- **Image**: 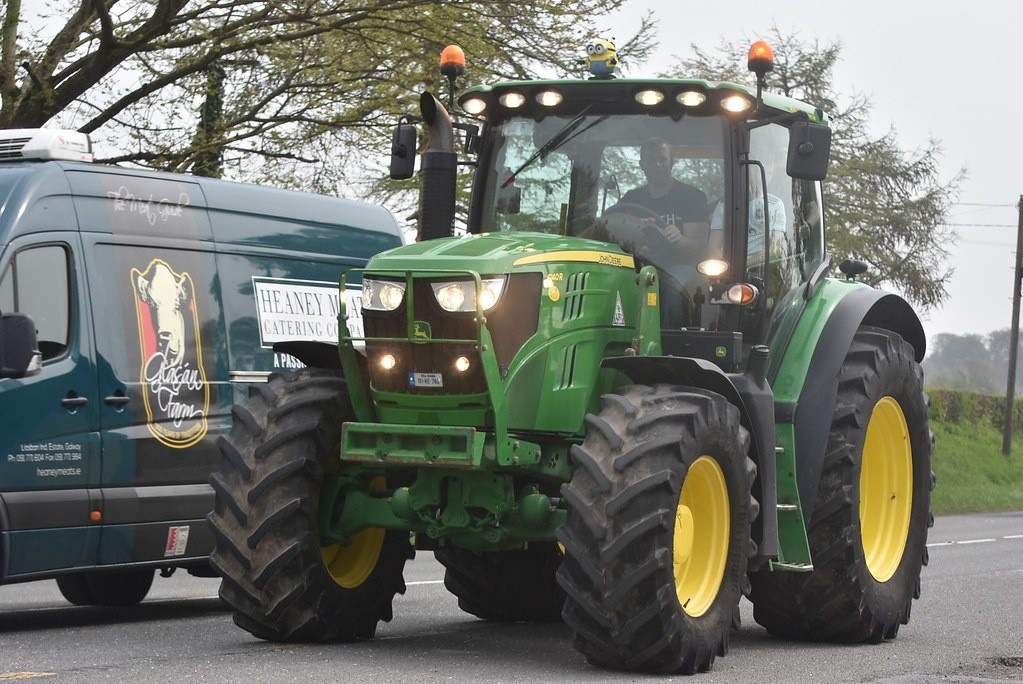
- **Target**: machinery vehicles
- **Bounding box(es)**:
[207,42,936,680]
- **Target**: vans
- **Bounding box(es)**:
[0,130,405,614]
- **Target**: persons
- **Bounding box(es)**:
[708,153,788,308]
[577,137,710,329]
